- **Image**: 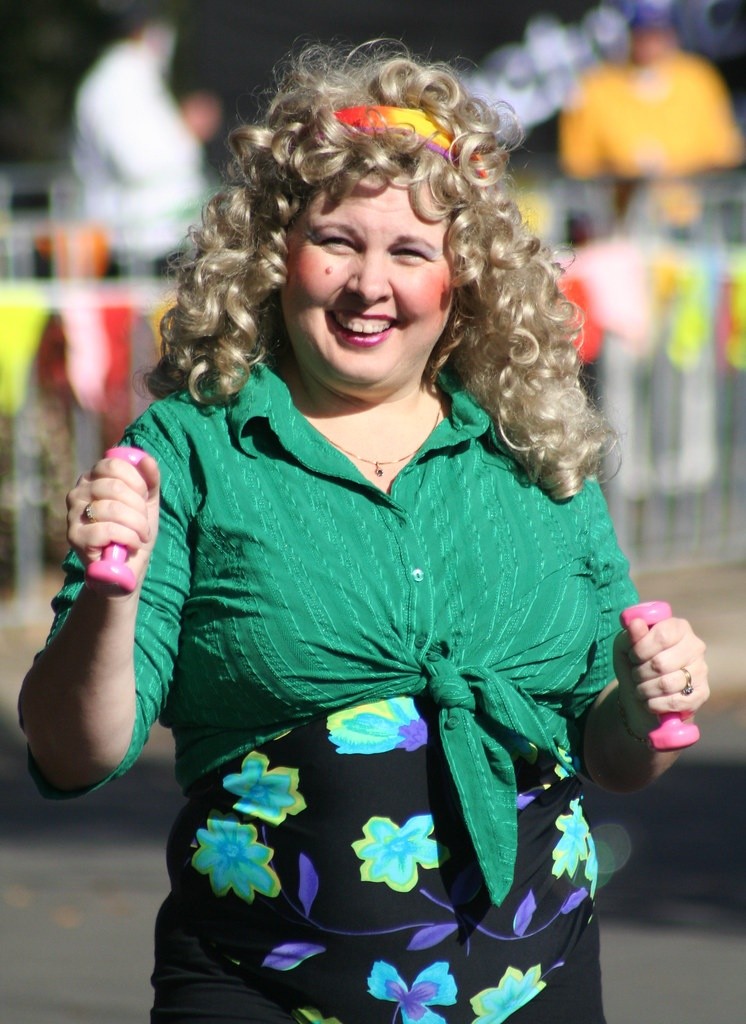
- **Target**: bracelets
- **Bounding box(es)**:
[617,695,648,742]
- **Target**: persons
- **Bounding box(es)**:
[17,39,711,1024]
[77,0,220,276]
[558,0,746,237]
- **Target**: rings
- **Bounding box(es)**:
[327,402,442,477]
[85,500,96,523]
[681,668,693,694]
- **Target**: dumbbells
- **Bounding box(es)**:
[82,443,155,600]
[620,595,705,752]
